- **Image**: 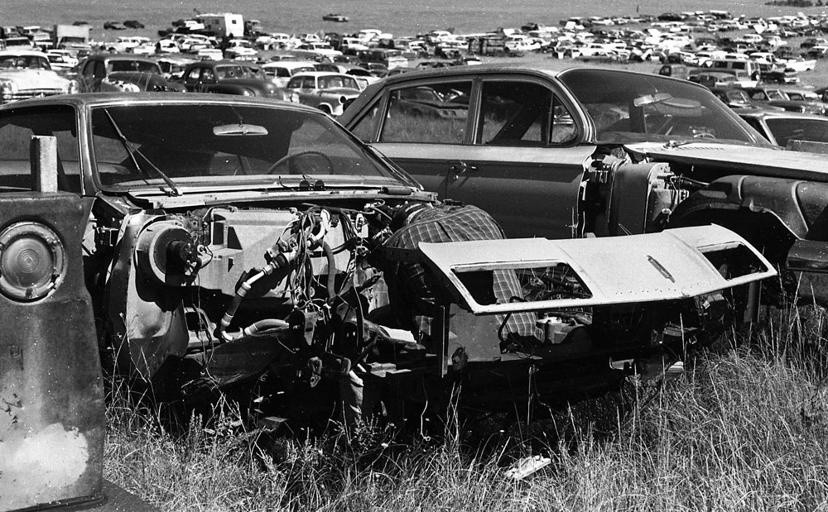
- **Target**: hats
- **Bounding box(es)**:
[389,202,427,234]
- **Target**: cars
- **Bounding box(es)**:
[339,66,825,300]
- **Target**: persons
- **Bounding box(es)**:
[375,198,539,366]
